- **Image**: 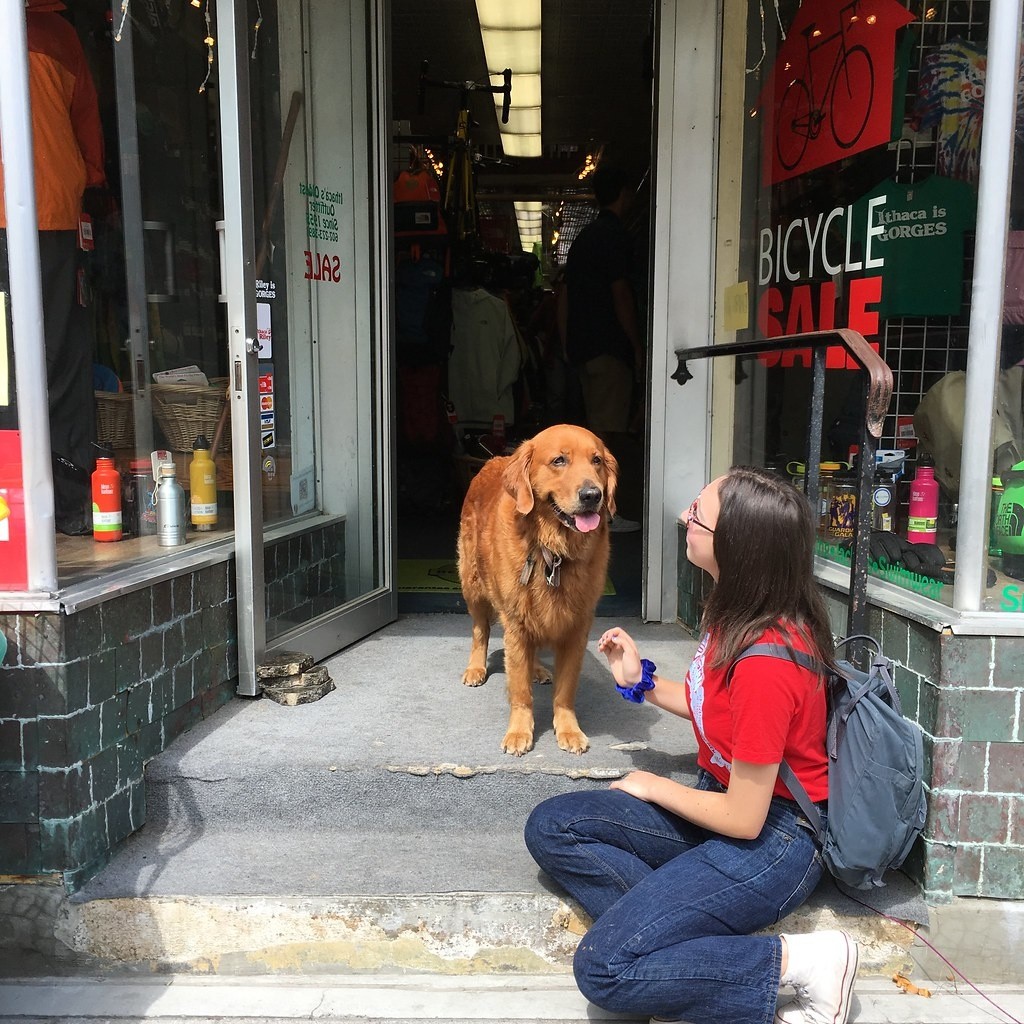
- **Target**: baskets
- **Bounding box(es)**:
[94,377,232,454]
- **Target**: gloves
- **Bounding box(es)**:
[839,530,946,575]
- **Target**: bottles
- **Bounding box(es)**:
[817,461,850,532]
[907,455,941,546]
[90,442,123,542]
[152,463,186,547]
[873,471,897,533]
[787,460,806,492]
[826,470,861,549]
[189,432,217,531]
[129,457,155,535]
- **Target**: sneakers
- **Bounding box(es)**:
[608,512,641,532]
[774,931,859,1024]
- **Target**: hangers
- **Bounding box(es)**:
[889,138,933,184]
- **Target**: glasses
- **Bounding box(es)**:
[686,486,714,533]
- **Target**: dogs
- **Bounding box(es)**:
[458,423,618,759]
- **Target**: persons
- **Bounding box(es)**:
[2,0,113,543]
[447,252,633,508]
[524,465,841,1024]
[566,169,638,533]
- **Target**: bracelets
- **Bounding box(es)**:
[615,660,655,703]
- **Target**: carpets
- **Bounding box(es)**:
[398,557,620,596]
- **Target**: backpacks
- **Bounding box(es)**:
[726,635,930,890]
[393,148,455,523]
